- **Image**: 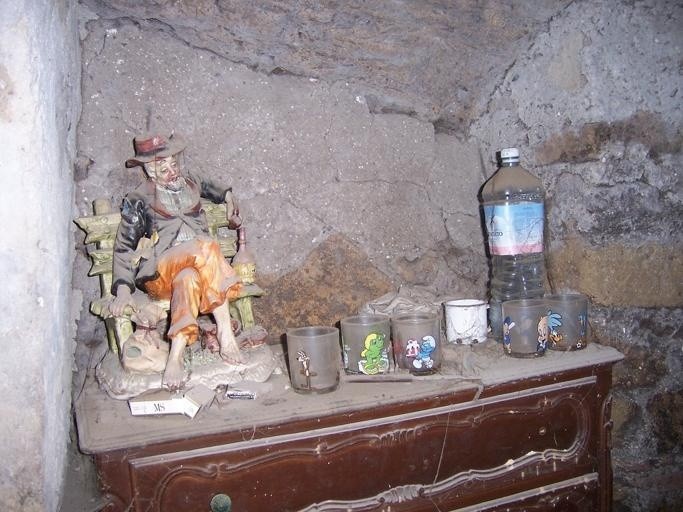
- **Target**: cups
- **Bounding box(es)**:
[502,299,548,358]
[441,298,492,346]
[285,325,341,394]
[393,313,443,376]
[338,315,392,375]
[544,293,589,353]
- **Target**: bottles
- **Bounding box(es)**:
[480,146,553,342]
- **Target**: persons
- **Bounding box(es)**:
[108,129,248,387]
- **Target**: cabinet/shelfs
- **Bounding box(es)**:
[76,340,626,512]
